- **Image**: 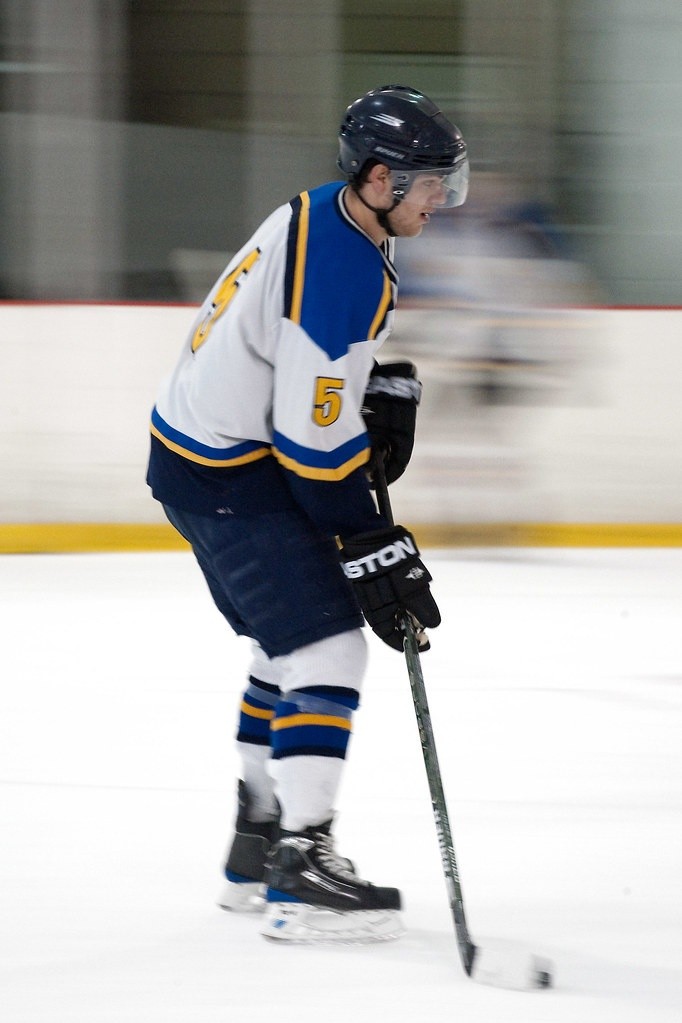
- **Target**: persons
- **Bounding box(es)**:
[146,84,471,949]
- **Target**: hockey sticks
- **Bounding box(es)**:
[370,454,553,996]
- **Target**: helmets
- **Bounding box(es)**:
[337,84,468,208]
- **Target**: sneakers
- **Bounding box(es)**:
[217,778,283,910]
[263,809,401,942]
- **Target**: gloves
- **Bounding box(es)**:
[361,359,422,490]
[337,525,441,653]
